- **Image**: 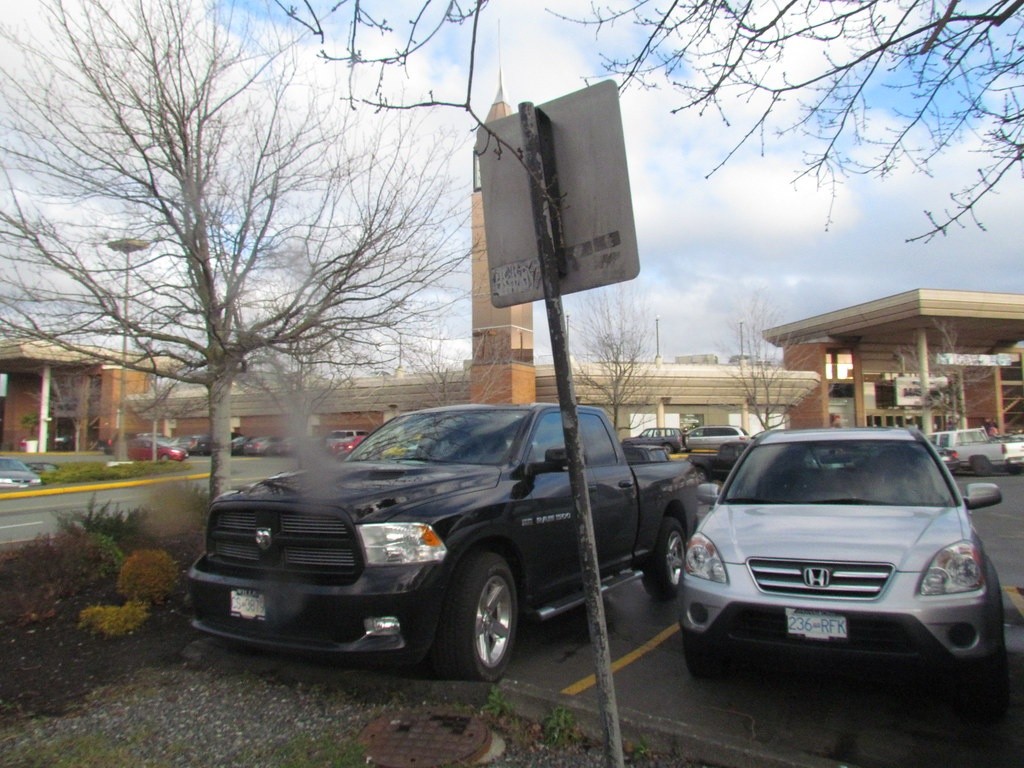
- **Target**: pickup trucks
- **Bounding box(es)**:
[923,426,1024,477]
[179,400,700,685]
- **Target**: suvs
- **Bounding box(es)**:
[622,427,683,454]
[682,425,749,454]
[676,422,1011,721]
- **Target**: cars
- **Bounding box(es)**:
[126,437,190,463]
[0,456,44,489]
[54,432,75,451]
[94,432,137,456]
[169,429,370,458]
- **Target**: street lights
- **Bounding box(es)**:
[105,237,152,462]
[655,314,661,358]
[740,320,744,360]
[566,312,571,351]
[395,331,403,368]
